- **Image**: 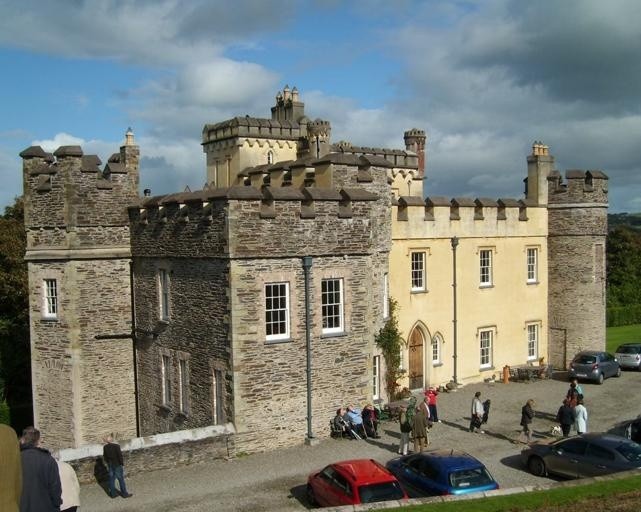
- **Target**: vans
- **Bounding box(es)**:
[615,343,641,371]
[569,350,621,385]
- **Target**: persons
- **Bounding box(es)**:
[0,423,80,512]
[398,388,441,456]
[470,392,483,433]
[555,378,588,437]
[481,398,490,424]
[334,402,381,439]
[104,433,133,498]
[517,400,535,443]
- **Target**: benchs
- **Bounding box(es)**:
[508,362,556,384]
[330,418,351,440]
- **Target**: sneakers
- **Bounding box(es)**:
[123,494,133,498]
[111,493,120,498]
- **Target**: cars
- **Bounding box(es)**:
[520,432,641,480]
[385,447,500,496]
[306,457,410,508]
